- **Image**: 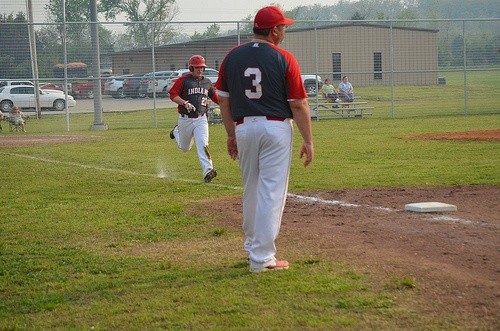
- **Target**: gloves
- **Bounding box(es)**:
[185,103,196,113]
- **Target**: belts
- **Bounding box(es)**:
[237,116,285,125]
[181,114,204,118]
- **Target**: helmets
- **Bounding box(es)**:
[189,55,206,66]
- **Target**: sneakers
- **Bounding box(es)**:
[204,169,217,182]
[246,256,289,272]
[170,125,178,139]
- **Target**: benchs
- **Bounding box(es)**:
[307,93,374,119]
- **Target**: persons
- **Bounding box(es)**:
[168,55,220,183]
[10,106,29,133]
[322,78,342,108]
[213,6,314,273]
[338,76,354,102]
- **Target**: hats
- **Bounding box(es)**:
[254,6,294,28]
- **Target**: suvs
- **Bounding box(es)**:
[104,73,132,98]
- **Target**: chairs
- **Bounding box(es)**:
[9,107,29,132]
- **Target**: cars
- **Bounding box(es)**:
[123,74,150,97]
[300,73,322,95]
[139,69,177,95]
[0,79,103,98]
[0,85,76,110]
[167,67,219,94]
